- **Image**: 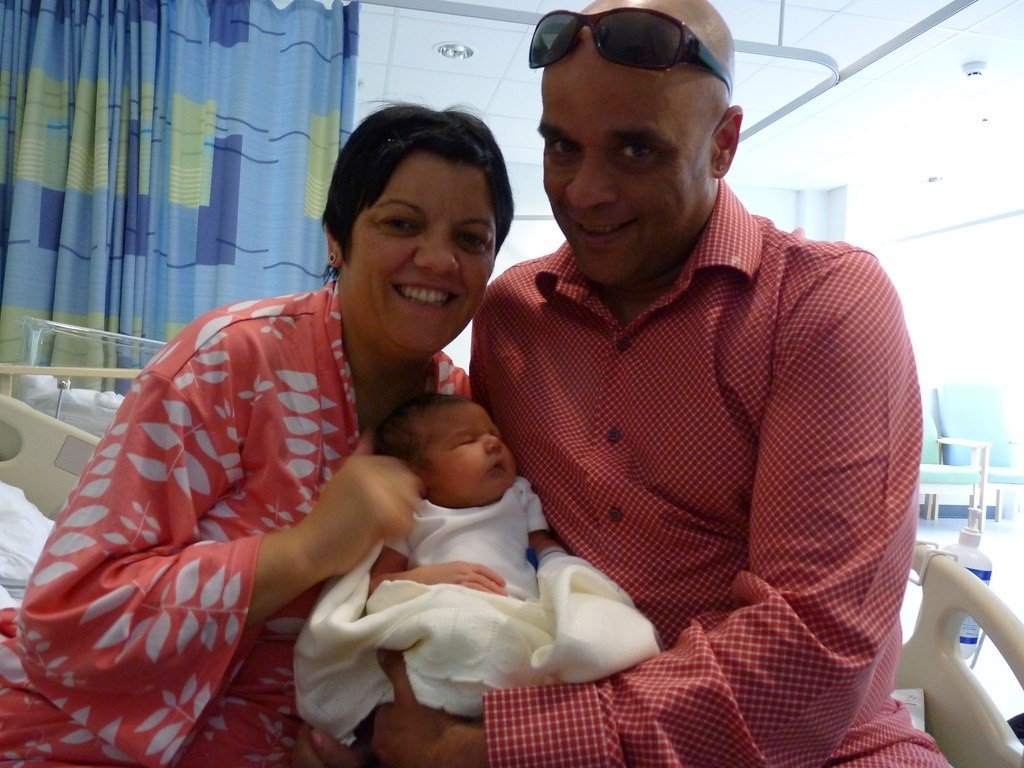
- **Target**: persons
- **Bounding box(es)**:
[1,105,516,768]
[370,1,948,768]
[295,394,660,747]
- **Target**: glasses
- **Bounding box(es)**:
[528,7,732,96]
